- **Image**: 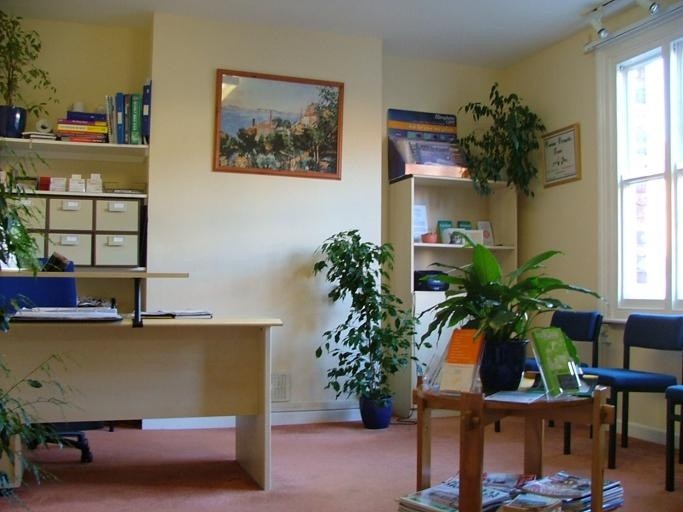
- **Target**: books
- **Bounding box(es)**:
[172,310,214,319]
[51,79,151,146]
[438,327,487,397]
[391,466,627,512]
[130,309,176,319]
[531,324,584,400]
[436,217,495,248]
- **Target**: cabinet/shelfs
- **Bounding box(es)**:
[384,172,521,418]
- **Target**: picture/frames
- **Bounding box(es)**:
[210,66,345,183]
[539,121,583,191]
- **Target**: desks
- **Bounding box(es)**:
[411,386,618,512]
[0,311,284,494]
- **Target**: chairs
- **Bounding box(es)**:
[492,309,683,493]
[1,254,97,465]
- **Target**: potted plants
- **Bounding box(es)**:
[0,8,58,137]
[312,227,419,429]
[419,231,604,398]
